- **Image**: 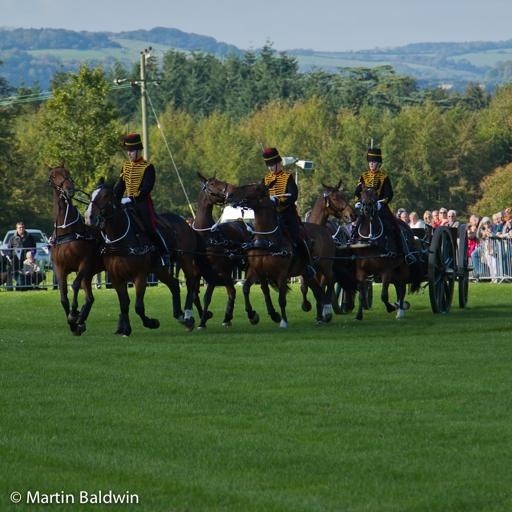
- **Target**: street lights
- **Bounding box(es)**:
[276,153,315,189]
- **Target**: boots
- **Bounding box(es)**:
[397,229,416,265]
[151,228,171,275]
[300,237,320,280]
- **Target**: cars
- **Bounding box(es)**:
[0,226,52,266]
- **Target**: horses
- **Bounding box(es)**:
[43,156,161,338]
[192,170,418,331]
[83,176,237,337]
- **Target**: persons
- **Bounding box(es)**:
[352,148,416,264]
[259,147,313,260]
[120,134,157,253]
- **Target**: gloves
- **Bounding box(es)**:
[121,197,132,205]
[354,202,361,209]
[271,196,276,205]
[376,200,381,211]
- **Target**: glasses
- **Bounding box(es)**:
[424,211,456,220]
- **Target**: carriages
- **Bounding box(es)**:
[47,130,478,336]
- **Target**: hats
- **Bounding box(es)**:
[262,147,283,167]
[122,133,145,151]
[366,148,383,163]
